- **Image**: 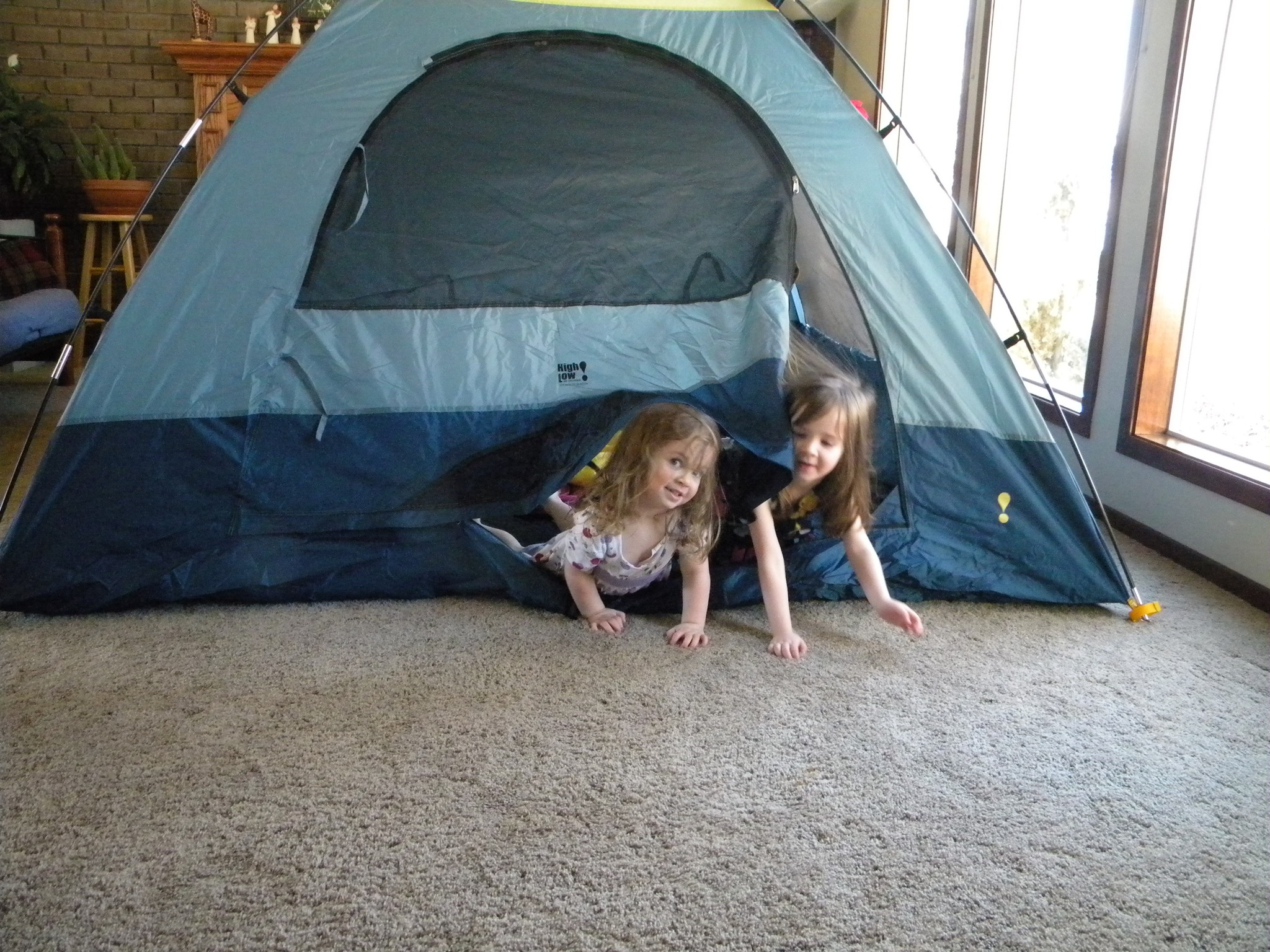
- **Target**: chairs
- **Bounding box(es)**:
[0,213,82,386]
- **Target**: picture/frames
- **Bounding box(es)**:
[287,0,339,33]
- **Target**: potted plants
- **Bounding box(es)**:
[69,123,152,216]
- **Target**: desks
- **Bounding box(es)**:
[73,215,153,367]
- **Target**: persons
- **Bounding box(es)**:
[314,19,323,31]
[291,16,302,45]
[245,17,256,43]
[470,327,924,660]
[265,5,282,43]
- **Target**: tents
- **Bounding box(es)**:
[0,0,1165,626]
